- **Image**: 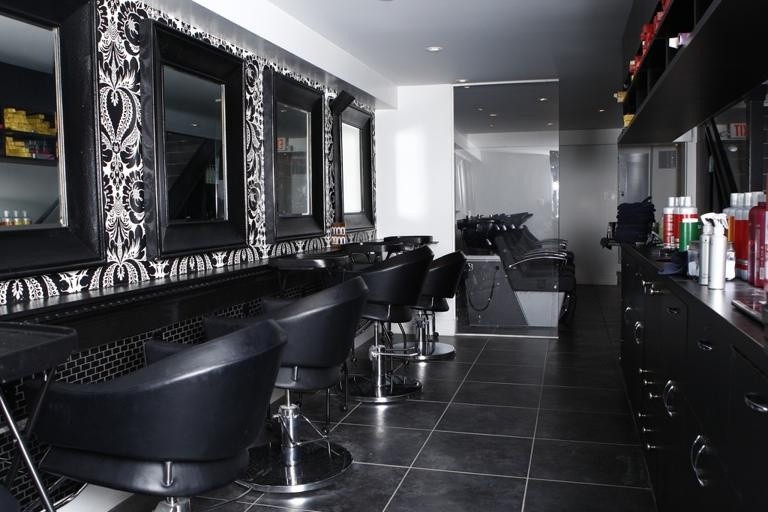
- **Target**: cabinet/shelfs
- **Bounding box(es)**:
[617,0,767,144]
[0,99,59,167]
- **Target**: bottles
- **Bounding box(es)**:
[720,190,768,291]
[662,195,699,254]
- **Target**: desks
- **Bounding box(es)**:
[0,319,79,512]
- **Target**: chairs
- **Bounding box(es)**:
[456,212,578,328]
